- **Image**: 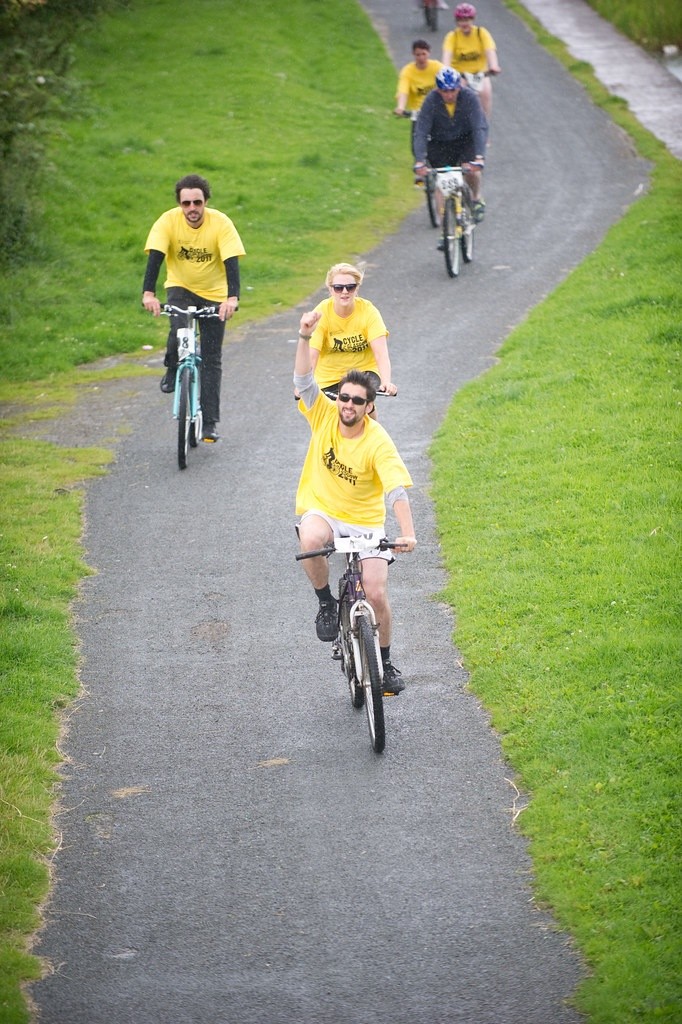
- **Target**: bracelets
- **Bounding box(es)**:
[298,330,312,340]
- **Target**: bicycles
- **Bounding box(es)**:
[293,535,407,754]
[424,1,440,31]
[461,71,498,93]
[394,110,441,227]
[425,164,477,277]
[324,386,396,397]
[145,303,237,469]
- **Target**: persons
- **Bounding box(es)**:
[293,311,417,692]
[412,66,488,251]
[441,3,502,147]
[395,39,446,186]
[293,263,398,420]
[141,174,246,442]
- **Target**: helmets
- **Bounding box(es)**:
[454,4,476,18]
[436,67,461,90]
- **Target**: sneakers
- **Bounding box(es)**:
[382,663,405,692]
[438,239,444,251]
[160,369,176,392]
[315,596,338,640]
[203,423,219,443]
[471,196,485,221]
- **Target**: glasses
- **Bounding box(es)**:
[331,284,357,292]
[179,200,204,208]
[338,392,370,405]
